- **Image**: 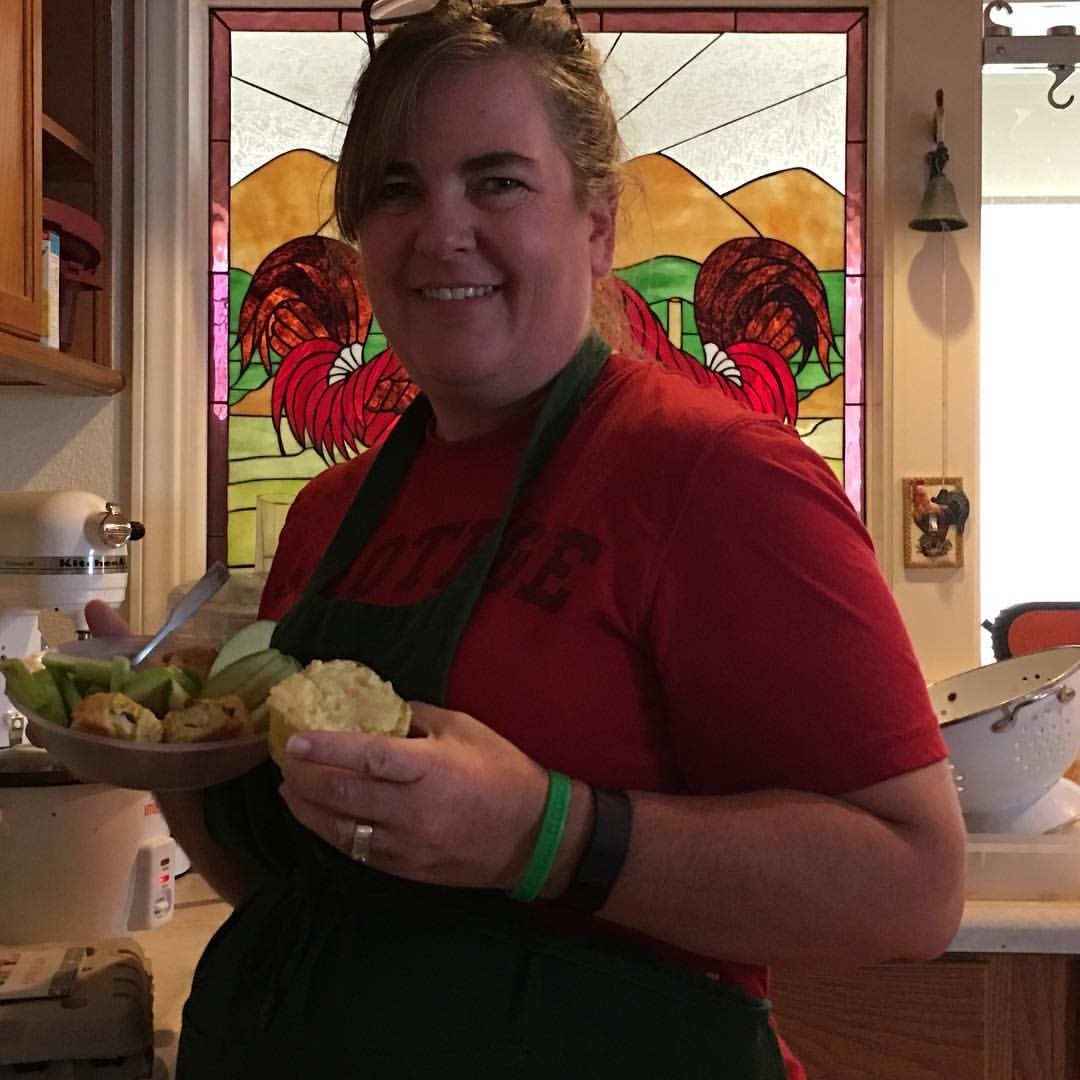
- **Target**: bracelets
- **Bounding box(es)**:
[511,773,575,902]
[561,781,633,919]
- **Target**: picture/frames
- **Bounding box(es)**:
[902,477,964,569]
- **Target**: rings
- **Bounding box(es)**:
[351,821,374,865]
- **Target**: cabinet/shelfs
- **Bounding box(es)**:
[1,0,124,397]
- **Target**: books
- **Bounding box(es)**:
[41,228,61,352]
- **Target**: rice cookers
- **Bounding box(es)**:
[0,743,179,941]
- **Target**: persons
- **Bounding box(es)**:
[26,1,968,1078]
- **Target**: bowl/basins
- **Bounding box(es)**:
[6,674,292,797]
